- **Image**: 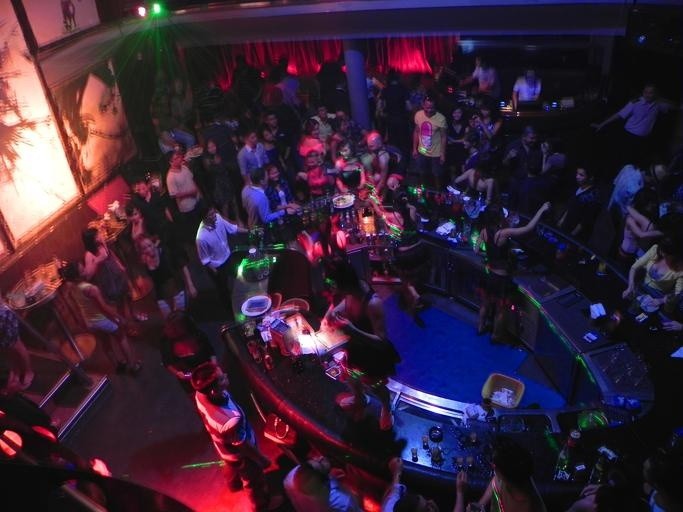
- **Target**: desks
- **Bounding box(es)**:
[86,175,184,298]
[9,258,99,366]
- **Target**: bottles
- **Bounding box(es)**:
[344,211,359,243]
[587,457,608,485]
[51,254,63,270]
[551,429,582,480]
[301,188,333,226]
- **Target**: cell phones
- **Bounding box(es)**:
[633,312,649,323]
[184,370,192,376]
[595,445,623,464]
[574,463,594,482]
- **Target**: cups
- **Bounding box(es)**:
[410,430,476,471]
[451,204,460,216]
[598,259,607,273]
[24,264,46,281]
[245,336,273,372]
[662,293,680,313]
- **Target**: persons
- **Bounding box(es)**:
[1,52,683,512]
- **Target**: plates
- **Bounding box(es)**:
[240,295,272,317]
[332,194,355,210]
